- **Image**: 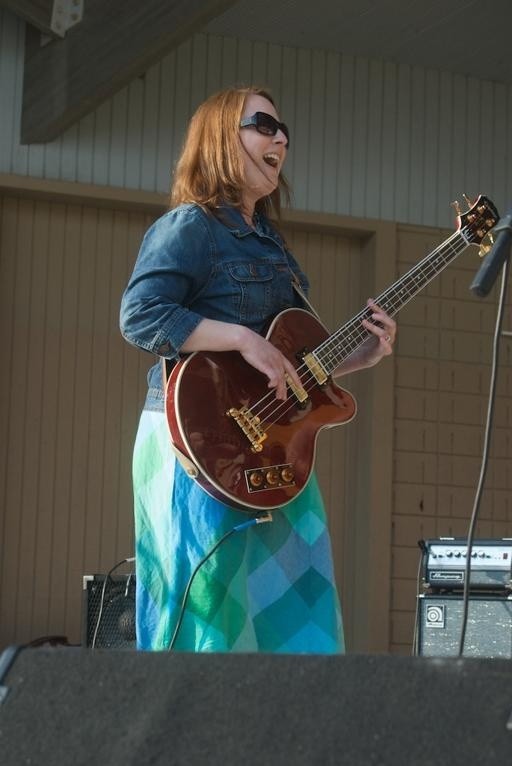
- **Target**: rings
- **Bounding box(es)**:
[385,335,392,344]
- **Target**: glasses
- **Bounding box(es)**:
[239,112,289,150]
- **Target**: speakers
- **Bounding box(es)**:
[80,573,136,651]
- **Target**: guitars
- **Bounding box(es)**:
[164,192,500,512]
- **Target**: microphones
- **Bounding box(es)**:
[464,213,511,298]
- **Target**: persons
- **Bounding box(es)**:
[119,86,397,653]
